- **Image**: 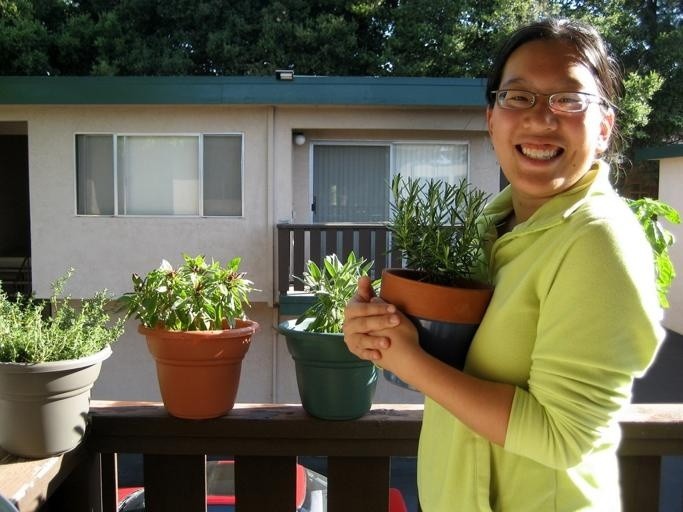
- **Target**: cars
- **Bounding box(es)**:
[117,459,408,512]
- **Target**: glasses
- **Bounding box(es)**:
[490,88,609,114]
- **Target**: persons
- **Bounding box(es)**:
[344,16,668,512]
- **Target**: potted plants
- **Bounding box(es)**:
[271,249,384,425]
[113,250,263,424]
[0,262,124,461]
[377,169,499,392]
